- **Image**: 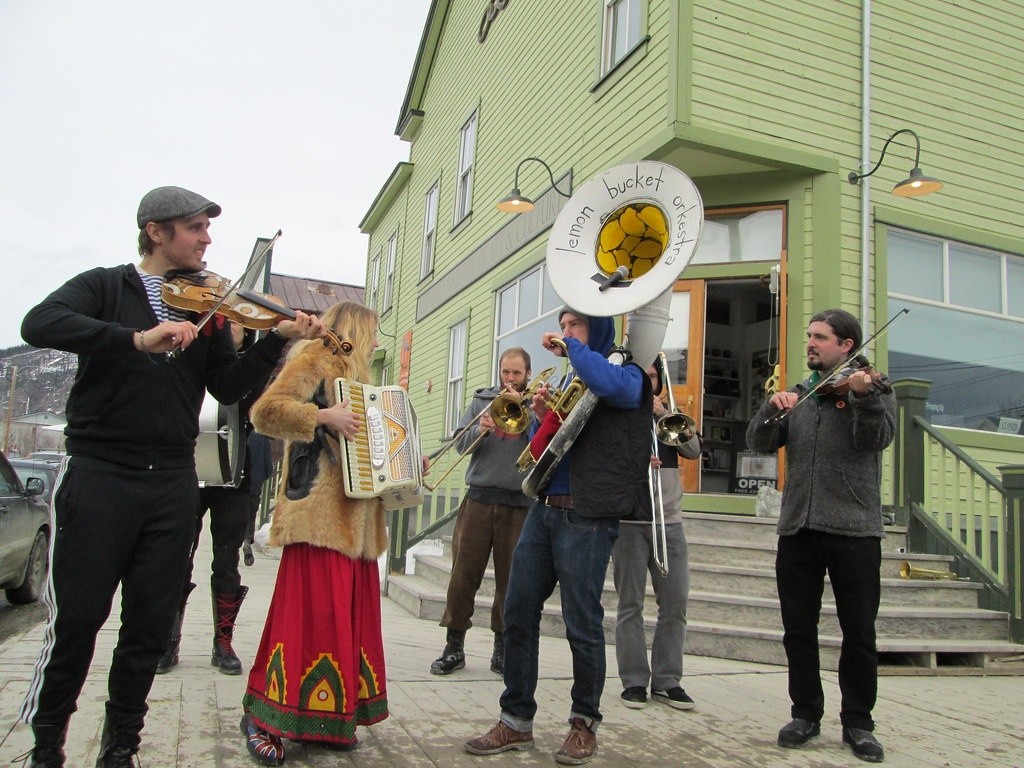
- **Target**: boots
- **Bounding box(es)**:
[431,628,467,675]
[490,630,504,677]
[211,585,249,674]
[155,582,198,674]
[96,724,141,768]
[12,724,65,768]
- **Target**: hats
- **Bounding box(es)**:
[558,304,591,332]
[137,186,221,231]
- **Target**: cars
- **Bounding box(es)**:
[0,451,52,603]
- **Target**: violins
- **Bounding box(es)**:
[160,267,354,358]
[814,359,894,397]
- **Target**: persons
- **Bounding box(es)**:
[20,186,328,768]
[612,355,701,709]
[463,305,654,766]
[155,320,275,675]
[745,309,897,763]
[429,347,533,678]
[239,301,431,766]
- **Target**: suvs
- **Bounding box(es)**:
[28,447,68,476]
[7,457,56,506]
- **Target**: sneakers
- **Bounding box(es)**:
[779,717,821,750]
[843,725,883,762]
[554,717,598,765]
[465,719,534,755]
[620,686,648,709]
[651,684,694,709]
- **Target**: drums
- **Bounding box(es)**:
[195,385,248,490]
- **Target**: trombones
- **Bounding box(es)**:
[647,351,696,576]
[421,366,557,492]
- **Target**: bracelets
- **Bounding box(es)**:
[141,330,148,354]
[271,328,287,340]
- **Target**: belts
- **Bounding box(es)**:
[542,493,574,510]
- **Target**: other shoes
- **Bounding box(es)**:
[290,736,356,752]
[242,544,254,566]
[239,709,285,766]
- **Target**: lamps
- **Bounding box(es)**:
[495,157,571,212]
[847,130,943,196]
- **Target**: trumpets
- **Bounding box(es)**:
[900,561,971,583]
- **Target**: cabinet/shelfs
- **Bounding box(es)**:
[702,353,746,474]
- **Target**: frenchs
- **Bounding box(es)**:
[515,160,704,495]
